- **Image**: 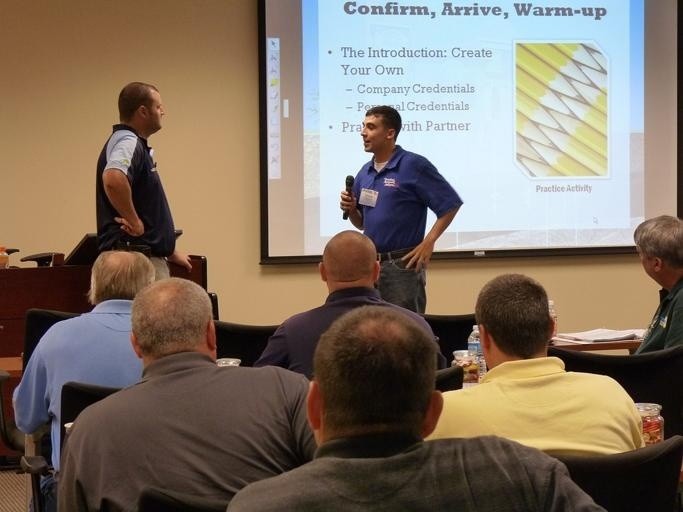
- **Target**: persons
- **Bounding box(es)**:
[423,273,645,455]
[93,81,192,279]
[339,105,463,316]
[250,230,447,380]
[631,215,683,355]
[52,276,304,506]
[13,249,159,510]
[225,305,606,512]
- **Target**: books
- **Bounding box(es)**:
[556,327,651,344]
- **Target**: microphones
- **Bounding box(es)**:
[342,176,356,223]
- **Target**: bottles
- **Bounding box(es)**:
[469,325,486,380]
[0,246,9,268]
[636,403,663,446]
[549,300,557,343]
[453,351,478,389]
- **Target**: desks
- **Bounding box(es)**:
[548,335,642,355]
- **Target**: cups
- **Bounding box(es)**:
[449,350,468,366]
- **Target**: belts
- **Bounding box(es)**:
[376,247,413,262]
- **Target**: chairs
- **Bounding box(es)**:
[208,319,296,368]
[20,383,127,511]
[550,434,683,512]
[137,487,217,511]
[415,312,483,370]
[542,343,683,443]
[19,306,88,380]
